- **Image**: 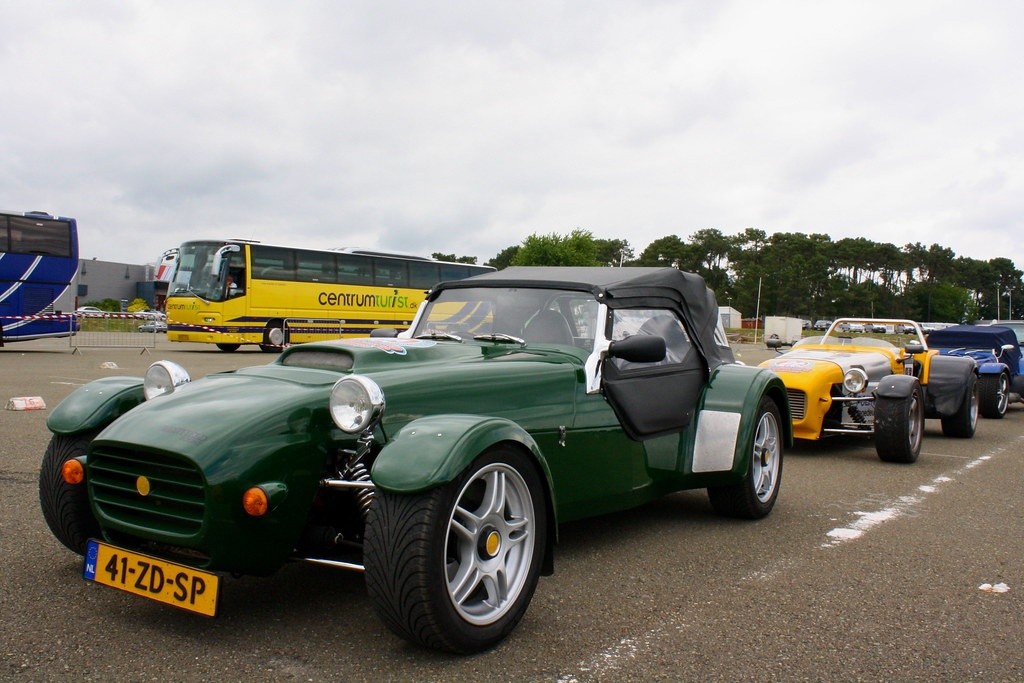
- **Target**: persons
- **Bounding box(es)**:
[227,277,238,289]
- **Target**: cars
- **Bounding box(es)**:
[924,319,1023,419]
[802,319,959,336]
[755,320,979,463]
[77,306,108,315]
[35,265,797,656]
[138,321,167,333]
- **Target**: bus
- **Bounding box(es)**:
[0,210,79,347]
[159,238,498,352]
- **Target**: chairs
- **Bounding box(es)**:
[520,309,575,344]
[619,315,692,371]
